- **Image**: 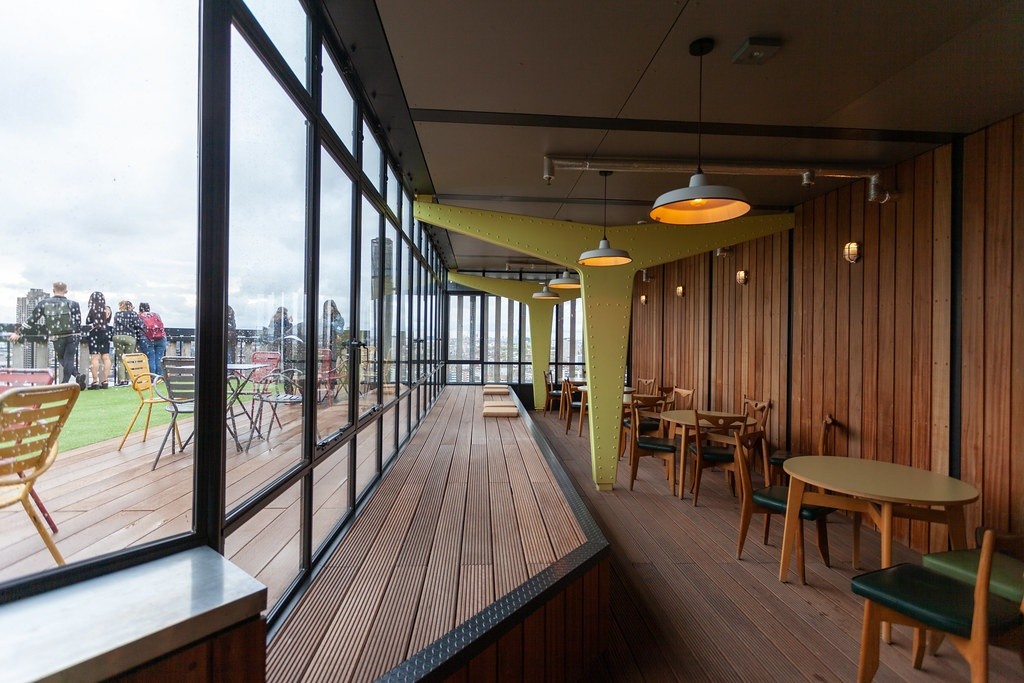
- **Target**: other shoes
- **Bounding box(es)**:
[61,380,68,384]
[99,380,108,389]
[76,374,86,390]
[88,381,99,390]
[115,381,129,387]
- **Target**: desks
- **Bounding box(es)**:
[566,375,588,384]
[177,364,272,454]
[622,393,673,467]
[658,410,757,500]
[779,455,981,645]
[575,384,636,437]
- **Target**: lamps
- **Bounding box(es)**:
[577,171,633,267]
[532,260,560,300]
[639,296,647,306]
[676,286,683,296]
[842,242,862,264]
[650,38,751,224]
[548,219,580,289]
[735,269,749,288]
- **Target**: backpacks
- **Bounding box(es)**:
[44,299,73,335]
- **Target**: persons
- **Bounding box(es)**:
[262,299,345,397]
[9,281,86,391]
[227,304,238,377]
[86,290,168,391]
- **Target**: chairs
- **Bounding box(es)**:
[621,394,668,458]
[628,402,677,495]
[770,414,833,494]
[541,370,567,419]
[655,383,677,411]
[0,346,395,567]
[671,385,695,410]
[912,526,1024,668]
[636,375,655,394]
[733,426,839,568]
[690,409,749,506]
[851,530,1024,683]
[740,394,774,429]
[563,379,589,436]
